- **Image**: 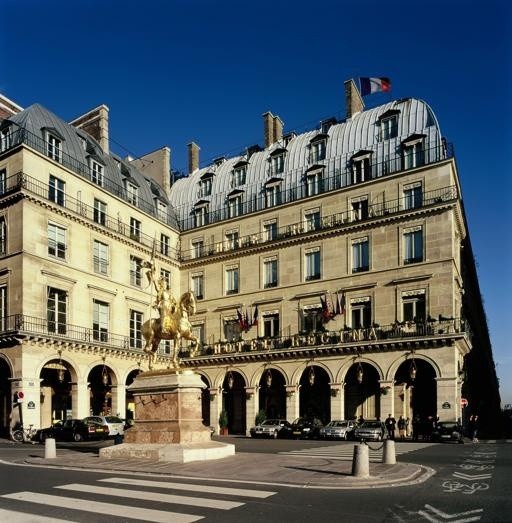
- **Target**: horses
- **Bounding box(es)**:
[141,288,200,370]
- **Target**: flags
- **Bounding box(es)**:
[235,302,260,330]
[357,75,392,98]
[317,288,348,319]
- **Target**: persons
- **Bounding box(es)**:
[406,416,410,435]
[468,412,483,441]
[411,412,440,440]
[189,312,449,356]
[377,414,382,421]
[354,415,360,424]
[358,413,366,424]
[149,269,177,334]
[397,414,408,439]
[385,413,397,438]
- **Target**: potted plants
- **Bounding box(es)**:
[219,408,229,436]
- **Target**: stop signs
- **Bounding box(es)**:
[461,399,468,409]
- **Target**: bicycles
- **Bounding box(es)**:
[11,424,39,444]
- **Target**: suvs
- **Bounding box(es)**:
[285,416,324,438]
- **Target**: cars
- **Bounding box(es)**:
[354,421,390,440]
[250,419,288,439]
[35,419,109,443]
[320,420,358,440]
[85,415,125,438]
[431,421,462,442]
